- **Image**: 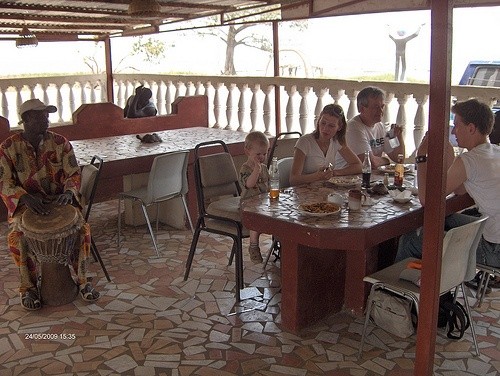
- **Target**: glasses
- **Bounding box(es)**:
[136,133,162,143]
[323,105,342,114]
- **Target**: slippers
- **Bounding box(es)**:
[464,278,492,294]
[20,284,42,311]
[78,281,101,302]
[474,270,500,288]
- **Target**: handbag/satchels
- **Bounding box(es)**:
[367,288,415,339]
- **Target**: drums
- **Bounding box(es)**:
[18,198,84,307]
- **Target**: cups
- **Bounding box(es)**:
[328,193,342,206]
[348,190,366,212]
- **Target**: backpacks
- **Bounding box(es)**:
[412,290,470,340]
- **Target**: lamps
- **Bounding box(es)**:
[16,19,39,47]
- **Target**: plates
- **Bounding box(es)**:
[378,165,410,173]
[328,176,363,186]
[297,204,342,218]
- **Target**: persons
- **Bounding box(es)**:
[239,130,272,264]
[123,84,158,119]
[335,86,405,172]
[288,104,363,187]
[393,99,500,269]
[0,99,101,310]
[384,23,424,82]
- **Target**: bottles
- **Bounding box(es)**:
[384,173,388,188]
[394,154,404,188]
[269,157,280,199]
[361,150,372,190]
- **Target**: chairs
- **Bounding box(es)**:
[184,140,252,302]
[263,132,302,270]
[360,213,500,356]
[115,149,195,256]
[79,154,112,283]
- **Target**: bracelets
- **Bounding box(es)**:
[414,155,427,163]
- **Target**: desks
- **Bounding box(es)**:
[69,126,277,231]
[242,169,475,335]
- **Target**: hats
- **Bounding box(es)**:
[19,99,57,116]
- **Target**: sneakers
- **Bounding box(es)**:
[248,246,263,264]
